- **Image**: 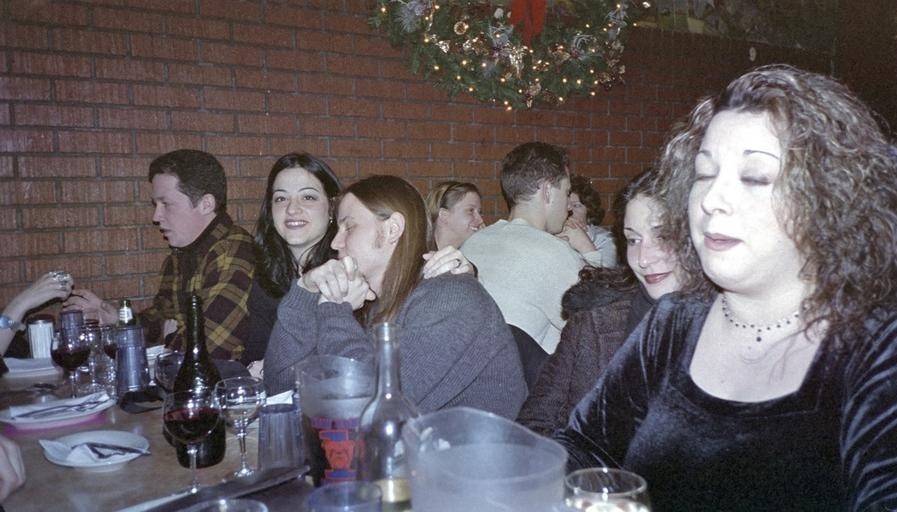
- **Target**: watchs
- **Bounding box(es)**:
[0,315,30,332]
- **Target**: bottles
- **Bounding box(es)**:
[26,317,56,360]
[171,293,228,469]
[115,299,135,325]
[359,320,415,511]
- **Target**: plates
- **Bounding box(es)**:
[44,430,150,475]
[0,396,117,432]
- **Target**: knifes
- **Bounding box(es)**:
[81,438,151,457]
[12,400,103,418]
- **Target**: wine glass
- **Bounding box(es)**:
[215,375,267,485]
[162,388,222,499]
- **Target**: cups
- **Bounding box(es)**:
[258,403,306,473]
[294,354,375,486]
[304,480,383,511]
[565,466,651,511]
[185,498,268,510]
[52,310,181,402]
[47,269,73,300]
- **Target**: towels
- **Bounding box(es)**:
[267,389,294,405]
[38,440,136,463]
[3,357,55,373]
[146,345,165,360]
[6,390,109,422]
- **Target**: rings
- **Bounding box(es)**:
[456,258,461,267]
[48,270,69,291]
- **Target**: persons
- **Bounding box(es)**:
[462,143,587,357]
[0,267,73,365]
[1,433,29,505]
[424,181,486,250]
[538,60,894,509]
[244,152,477,364]
[553,175,616,269]
[263,175,530,439]
[507,164,694,440]
[60,148,259,362]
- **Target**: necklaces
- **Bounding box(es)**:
[713,291,822,342]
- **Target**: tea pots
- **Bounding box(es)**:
[401,405,568,511]
